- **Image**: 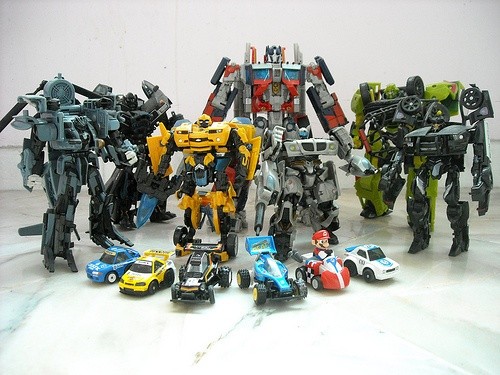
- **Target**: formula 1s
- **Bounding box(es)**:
[235,235,308,306]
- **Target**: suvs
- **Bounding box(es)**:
[169,242,233,304]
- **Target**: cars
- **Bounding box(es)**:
[287,247,351,291]
[118,249,178,297]
[85,245,141,284]
[343,243,401,283]
